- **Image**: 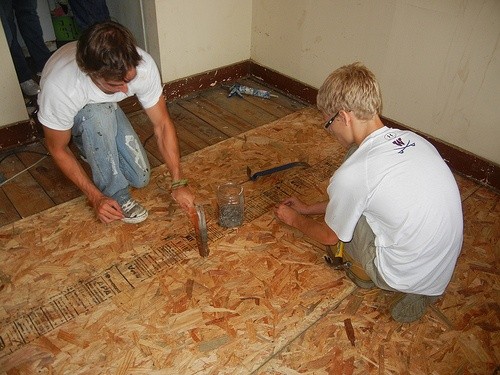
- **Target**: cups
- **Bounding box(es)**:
[215,182,244,228]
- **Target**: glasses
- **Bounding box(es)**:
[322,108,351,133]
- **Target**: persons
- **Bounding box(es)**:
[273,61,464,324]
[36,21,196,225]
[0,0,113,95]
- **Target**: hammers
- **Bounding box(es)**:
[324,241,352,270]
[186,205,210,256]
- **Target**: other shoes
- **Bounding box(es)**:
[388,284,446,323]
[327,243,376,289]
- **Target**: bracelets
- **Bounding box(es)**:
[171,179,190,190]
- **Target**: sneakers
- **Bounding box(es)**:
[20,79,39,95]
[116,197,148,223]
